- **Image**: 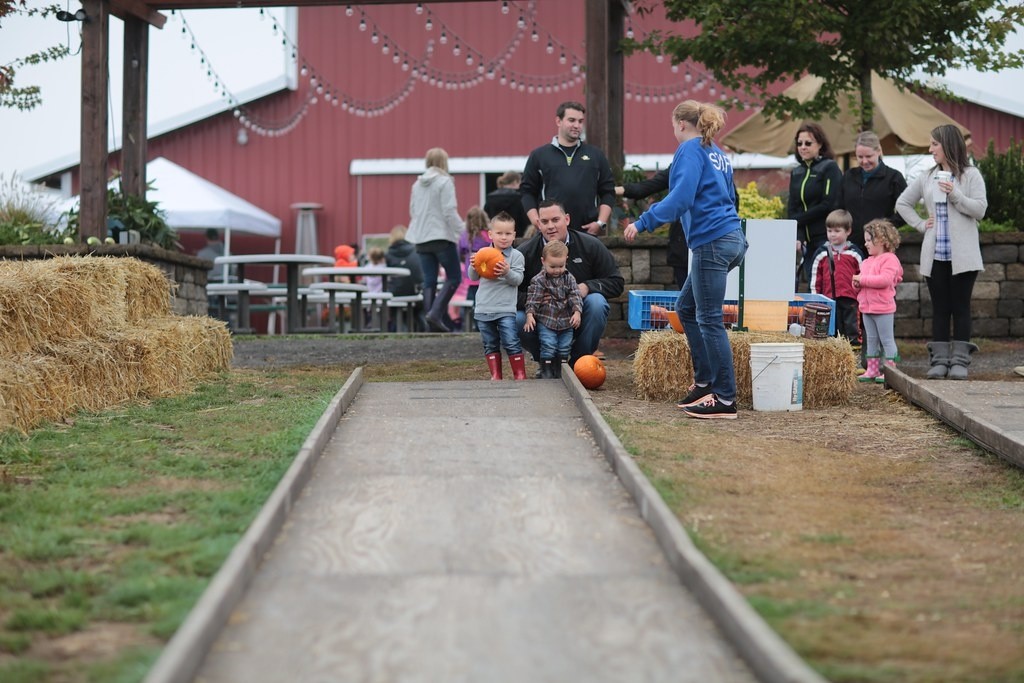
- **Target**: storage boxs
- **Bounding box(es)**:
[629,290,835,336]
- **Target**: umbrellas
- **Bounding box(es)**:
[719,50,972,171]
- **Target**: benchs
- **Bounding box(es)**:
[207,283,479,336]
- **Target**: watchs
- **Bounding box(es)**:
[598,221,607,230]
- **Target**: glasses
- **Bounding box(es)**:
[796,140,816,146]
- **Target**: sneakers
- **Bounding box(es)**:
[683,396,738,418]
[677,382,713,407]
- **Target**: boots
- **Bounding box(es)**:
[508,352,527,381]
[950,341,980,378]
[485,352,503,381]
[856,355,881,381]
[923,341,949,381]
[875,357,899,382]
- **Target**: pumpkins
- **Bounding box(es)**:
[573,355,607,390]
[788,296,805,324]
[473,247,504,279]
[650,306,670,329]
[722,304,739,325]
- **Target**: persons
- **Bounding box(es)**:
[196,228,237,283]
[523,239,583,377]
[458,206,492,330]
[517,197,627,373]
[334,245,363,283]
[386,224,425,332]
[405,148,467,331]
[363,248,387,291]
[616,99,748,419]
[896,124,988,381]
[810,209,863,349]
[852,219,898,382]
[787,122,844,280]
[484,169,529,238]
[837,130,908,257]
[468,211,527,380]
[519,101,615,358]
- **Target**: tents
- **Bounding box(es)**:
[68,156,282,337]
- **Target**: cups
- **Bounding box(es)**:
[938,171,951,183]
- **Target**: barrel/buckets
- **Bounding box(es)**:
[749,341,805,412]
[801,303,832,340]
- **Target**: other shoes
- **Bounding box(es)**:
[555,354,567,377]
[426,313,449,333]
[855,368,865,375]
[535,360,542,377]
[542,357,554,379]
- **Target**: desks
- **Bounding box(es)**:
[302,267,410,333]
[215,256,335,336]
